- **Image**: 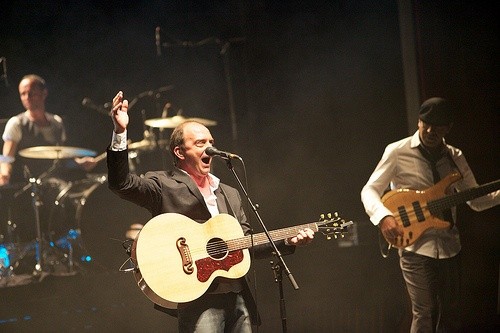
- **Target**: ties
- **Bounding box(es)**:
[418,145,443,184]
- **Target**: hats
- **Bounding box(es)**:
[418,97,456,127]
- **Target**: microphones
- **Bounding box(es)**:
[204,146,240,159]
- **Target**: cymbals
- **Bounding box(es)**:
[19,146,96,161]
[145,116,219,128]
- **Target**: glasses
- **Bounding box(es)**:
[194,138,214,147]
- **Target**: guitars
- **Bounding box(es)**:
[381,173,500,249]
[131,211,354,311]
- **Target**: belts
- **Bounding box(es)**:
[400,250,414,257]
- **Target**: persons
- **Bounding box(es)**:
[360,97,500,333]
[105,91,314,333]
[0,74,96,186]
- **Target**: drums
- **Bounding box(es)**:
[0,178,80,253]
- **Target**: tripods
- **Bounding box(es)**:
[3,160,81,283]
